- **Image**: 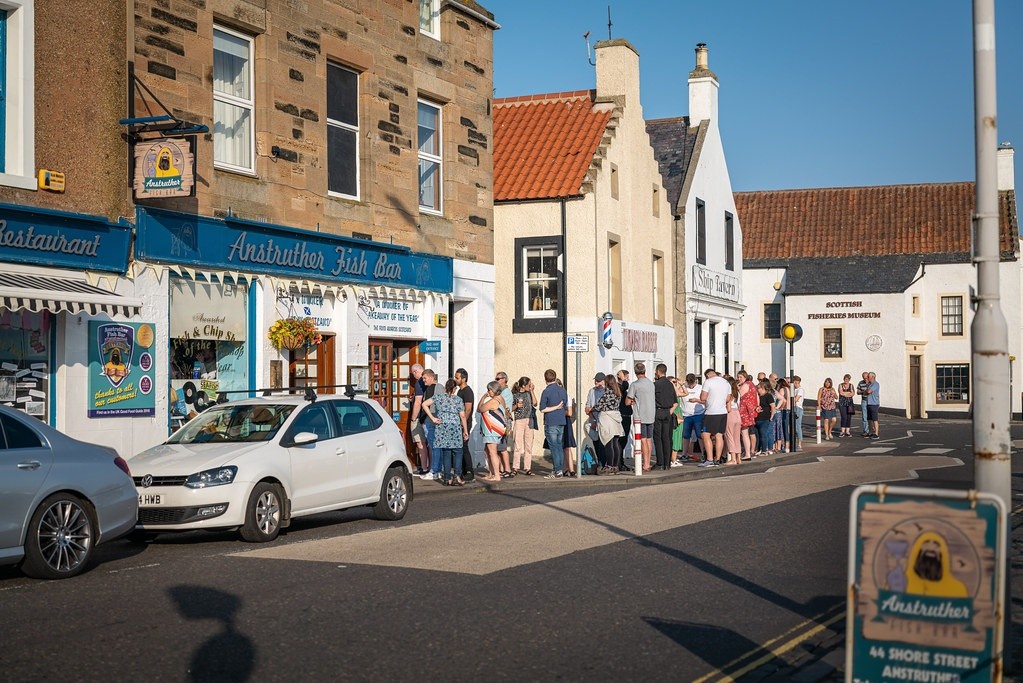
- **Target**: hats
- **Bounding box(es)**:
[593,372,605,382]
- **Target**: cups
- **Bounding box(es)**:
[518,399,523,404]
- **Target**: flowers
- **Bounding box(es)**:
[268,317,323,352]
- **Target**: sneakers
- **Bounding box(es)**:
[542,471,563,479]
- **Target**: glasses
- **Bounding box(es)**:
[495,377,504,381]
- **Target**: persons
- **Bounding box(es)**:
[584,370,634,476]
[455,368,475,480]
[667,375,689,467]
[510,377,538,477]
[539,369,568,479]
[650,364,678,470]
[818,378,838,441]
[838,374,855,438]
[411,364,445,482]
[856,372,880,439]
[422,379,469,486]
[625,363,656,472]
[477,381,505,481]
[678,373,707,463]
[495,372,514,478]
[201,343,216,374]
[555,378,575,477]
[697,367,804,467]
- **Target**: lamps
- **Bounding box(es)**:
[602,312,613,349]
[528,272,548,310]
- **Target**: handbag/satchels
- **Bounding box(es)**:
[581,444,598,475]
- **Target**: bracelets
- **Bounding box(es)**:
[411,419,416,421]
[681,383,685,386]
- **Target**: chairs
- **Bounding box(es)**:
[241,407,273,441]
[306,409,329,440]
[343,412,364,432]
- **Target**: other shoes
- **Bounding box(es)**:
[839,432,852,438]
[481,468,535,481]
[825,434,834,441]
[670,453,752,468]
[861,431,868,436]
[563,470,575,477]
[755,445,802,456]
[864,434,880,440]
[651,464,671,471]
[598,465,620,476]
[413,468,474,486]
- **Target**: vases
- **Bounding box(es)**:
[282,336,304,351]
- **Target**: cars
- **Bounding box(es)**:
[0,403,139,581]
[126,383,414,543]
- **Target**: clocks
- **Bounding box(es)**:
[866,335,882,350]
